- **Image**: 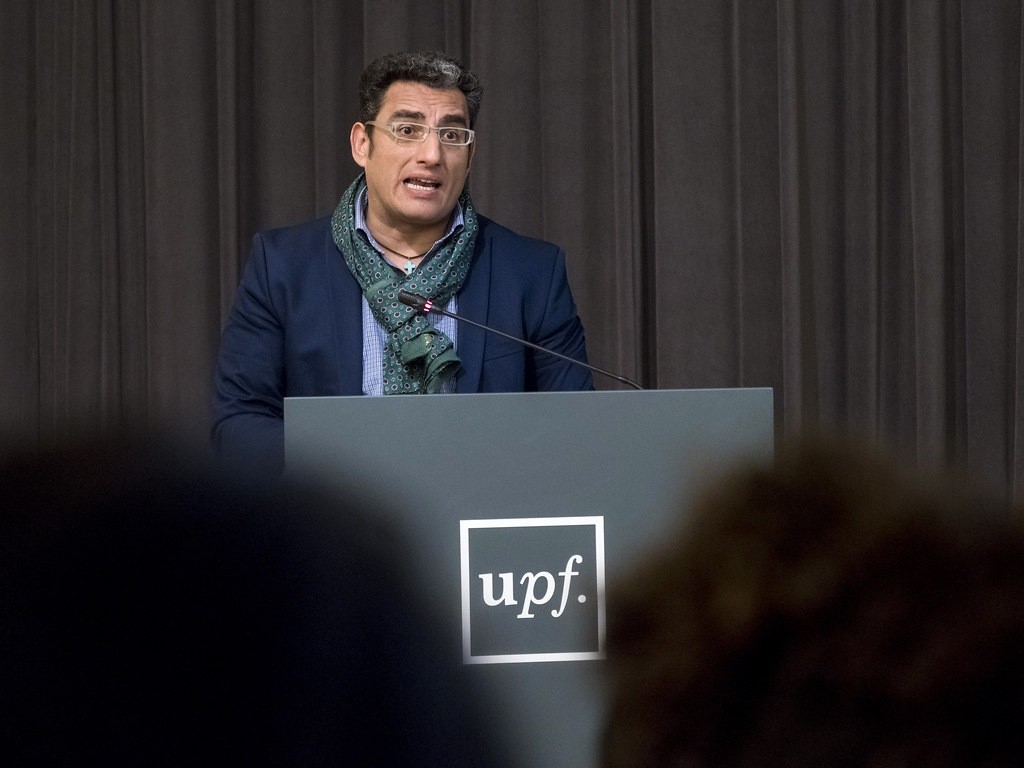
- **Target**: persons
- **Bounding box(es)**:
[599,442,1024,768]
[0,406,507,768]
[210,52,595,486]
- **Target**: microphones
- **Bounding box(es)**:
[398,290,645,391]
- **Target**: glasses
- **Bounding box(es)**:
[365,121,475,146]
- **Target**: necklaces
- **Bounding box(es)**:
[371,218,451,275]
